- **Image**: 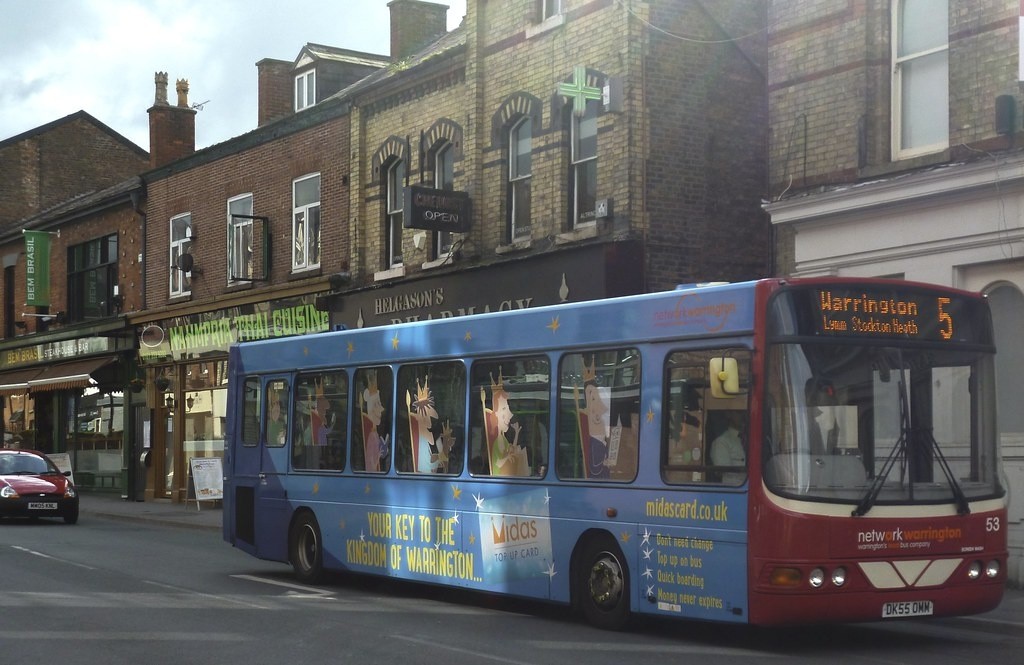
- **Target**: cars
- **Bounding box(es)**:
[0,448,79,524]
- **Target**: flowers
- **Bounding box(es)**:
[130,378,146,386]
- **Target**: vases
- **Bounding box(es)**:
[130,386,143,393]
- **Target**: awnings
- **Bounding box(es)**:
[0,357,127,395]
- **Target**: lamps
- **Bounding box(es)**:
[15,322,28,330]
[166,394,178,412]
[184,395,193,412]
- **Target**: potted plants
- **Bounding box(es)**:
[154,374,169,391]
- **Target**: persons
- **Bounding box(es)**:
[710,401,772,486]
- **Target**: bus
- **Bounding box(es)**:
[222,273,1009,635]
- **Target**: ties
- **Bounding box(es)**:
[739,433,747,453]
